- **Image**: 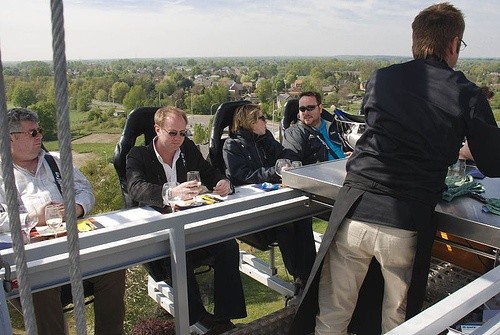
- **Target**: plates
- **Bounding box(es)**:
[35,222,67,236]
[0,230,13,243]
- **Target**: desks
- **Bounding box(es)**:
[0,180,309,257]
[281,156,500,251]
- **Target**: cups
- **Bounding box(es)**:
[444,156,466,186]
[292,161,302,168]
[275,158,291,178]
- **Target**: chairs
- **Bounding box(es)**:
[59,281,94,312]
[114,106,223,280]
[210,101,278,277]
[283,101,333,127]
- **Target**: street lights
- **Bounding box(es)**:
[266,98,275,131]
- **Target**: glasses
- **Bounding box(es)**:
[9,128,42,137]
[164,129,187,137]
[258,116,266,122]
[298,105,319,112]
[459,38,468,51]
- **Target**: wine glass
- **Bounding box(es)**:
[187,171,203,207]
[18,204,39,245]
[162,182,182,213]
[44,205,64,238]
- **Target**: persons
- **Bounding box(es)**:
[0,108,126,335]
[283,91,353,165]
[126,105,247,335]
[222,104,317,284]
[290,1,500,335]
